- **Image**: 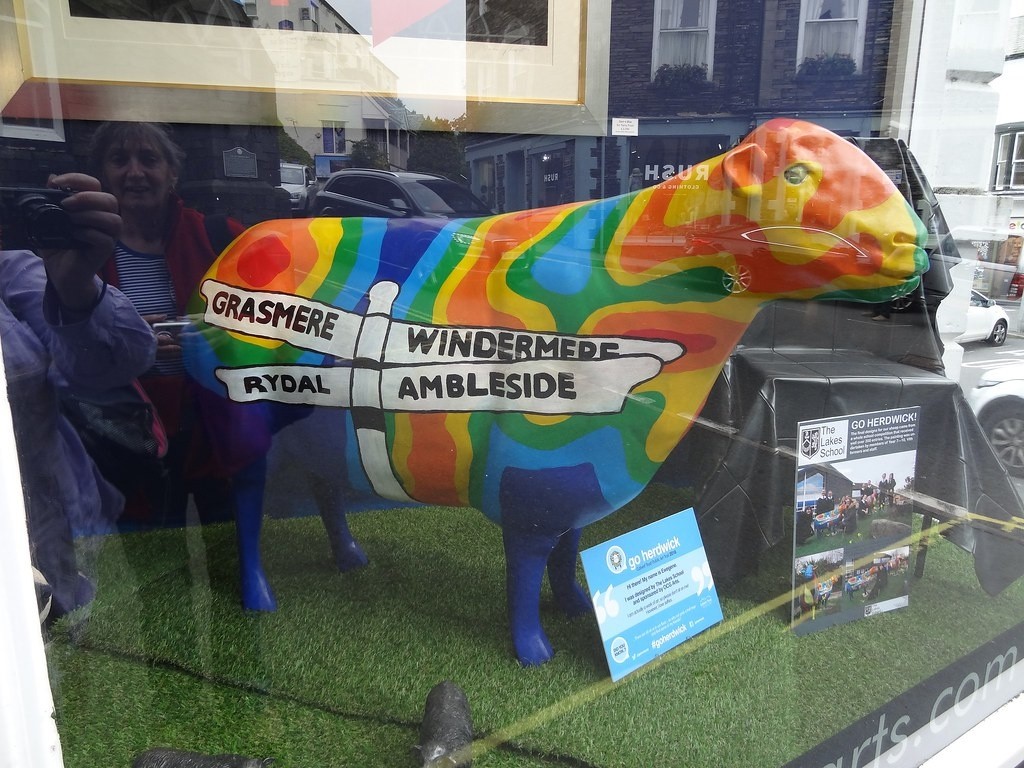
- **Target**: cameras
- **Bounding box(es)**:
[152,320,192,342]
[0,185,95,251]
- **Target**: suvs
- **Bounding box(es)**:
[309,168,498,224]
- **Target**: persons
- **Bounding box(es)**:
[75,117,317,731]
[796,469,904,611]
[222,156,295,232]
[0,169,161,651]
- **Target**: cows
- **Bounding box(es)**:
[180,119,930,668]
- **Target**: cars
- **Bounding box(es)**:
[937,285,1009,347]
[969,358,1023,479]
[275,161,318,215]
